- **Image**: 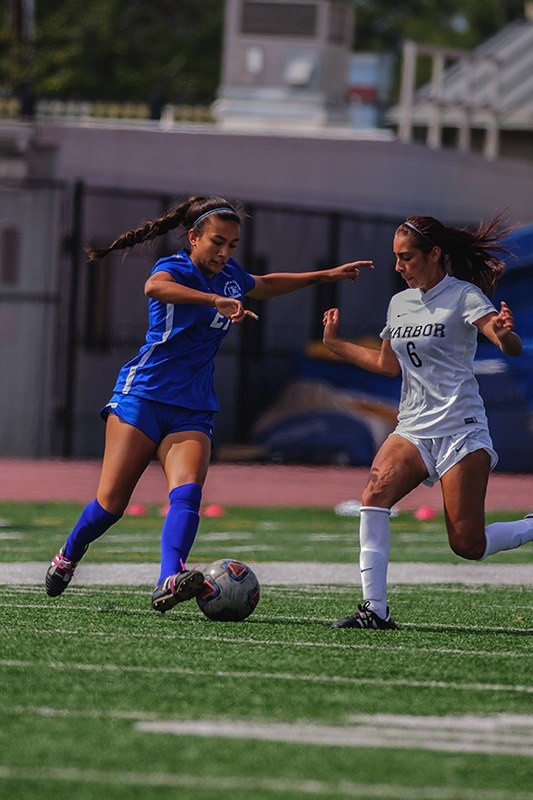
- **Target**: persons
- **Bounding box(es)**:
[46,196,375,612]
[323,215,532,629]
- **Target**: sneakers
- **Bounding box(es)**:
[332,599,399,629]
[46,540,90,597]
[151,558,205,615]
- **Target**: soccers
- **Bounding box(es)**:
[196,558,261,620]
